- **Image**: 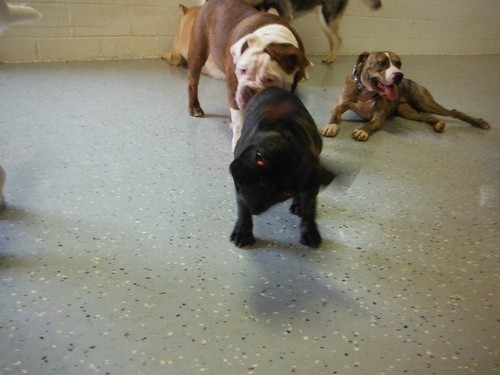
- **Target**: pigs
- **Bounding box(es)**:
[242,0,383,63]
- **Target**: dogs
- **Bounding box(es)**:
[160,3,226,81]
[229,85,335,249]
[319,50,492,142]
[187,0,315,153]
